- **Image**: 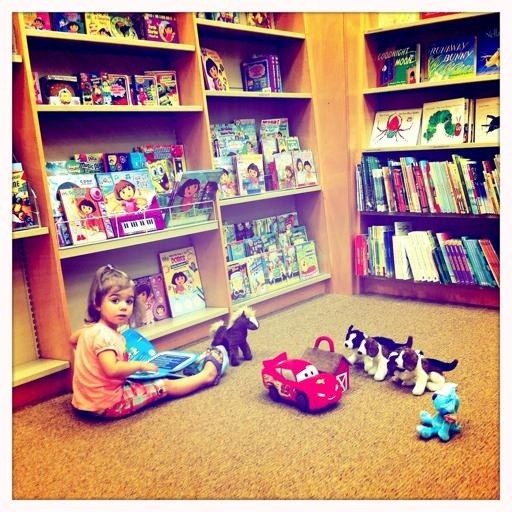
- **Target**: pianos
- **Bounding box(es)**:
[109,211,164,239]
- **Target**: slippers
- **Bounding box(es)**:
[202,345,230,386]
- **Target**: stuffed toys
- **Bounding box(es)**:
[416,385,462,442]
[206,304,261,368]
[344,324,414,368]
[356,337,425,382]
[389,348,458,396]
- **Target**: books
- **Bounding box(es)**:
[23,12,180,44]
[201,47,229,90]
[45,144,223,246]
[241,54,282,92]
[222,212,318,303]
[32,71,181,106]
[210,117,320,199]
[129,247,206,328]
[11,163,35,230]
[353,221,499,286]
[376,21,500,87]
[119,326,199,380]
[370,97,499,148]
[198,12,276,29]
[354,153,500,214]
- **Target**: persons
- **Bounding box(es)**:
[66,261,228,422]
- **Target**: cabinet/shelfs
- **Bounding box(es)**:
[359,12,499,310]
